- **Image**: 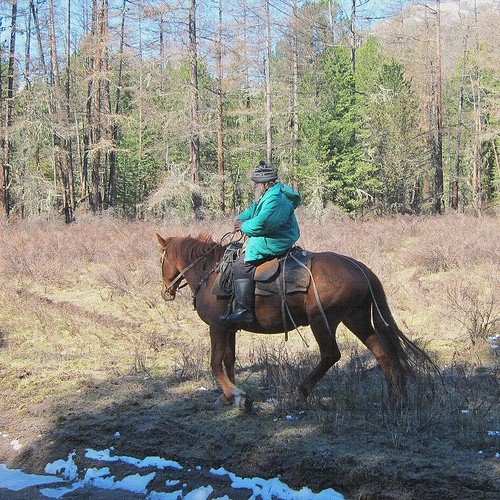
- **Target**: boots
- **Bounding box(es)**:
[218,279,254,322]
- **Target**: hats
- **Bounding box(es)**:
[251,160,278,183]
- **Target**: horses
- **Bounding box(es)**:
[156,230,444,414]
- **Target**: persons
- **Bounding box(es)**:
[219,160,301,323]
